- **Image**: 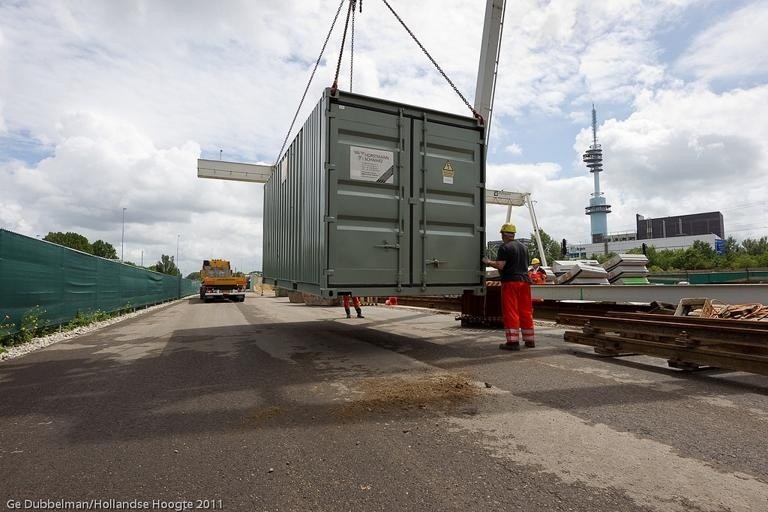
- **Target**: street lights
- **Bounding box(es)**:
[141,250,144,266]
[177,234,180,277]
[122,207,127,261]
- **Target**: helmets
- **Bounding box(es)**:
[500,223,516,233]
[531,258,540,264]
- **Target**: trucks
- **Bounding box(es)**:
[199,259,251,302]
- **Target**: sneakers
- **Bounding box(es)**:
[525,341,535,347]
[499,342,520,351]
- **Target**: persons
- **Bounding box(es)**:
[342,295,365,318]
[482,223,535,351]
[527,257,546,285]
